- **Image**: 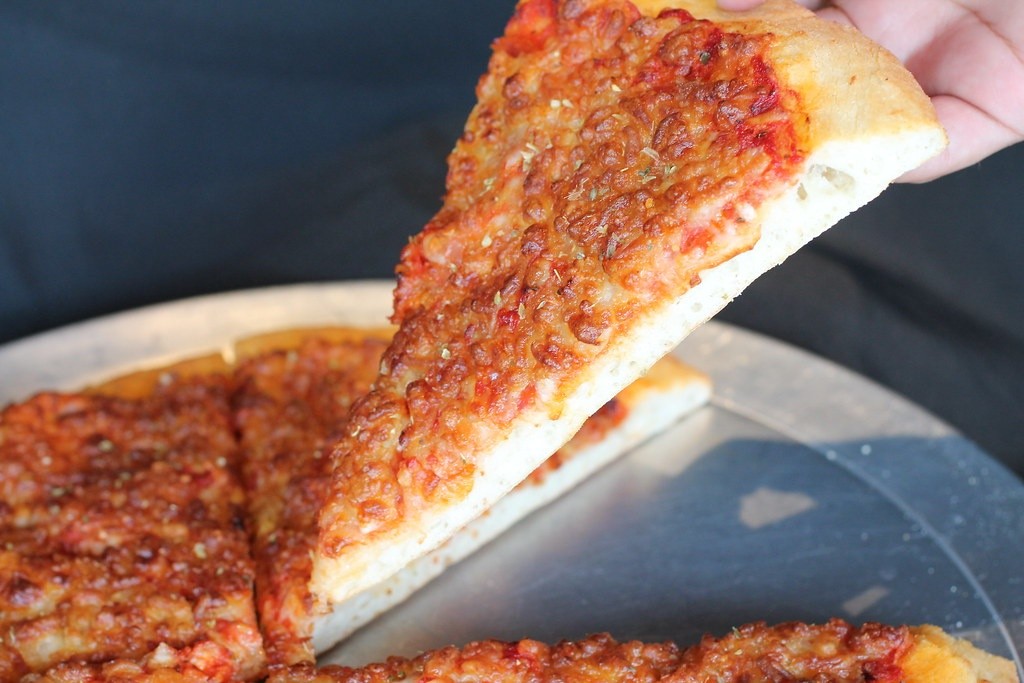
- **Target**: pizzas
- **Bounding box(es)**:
[0,328,1024,683]
[310,0,948,612]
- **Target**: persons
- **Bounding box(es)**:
[718,0,1024,185]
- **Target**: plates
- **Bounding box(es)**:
[2,275,1024,683]
